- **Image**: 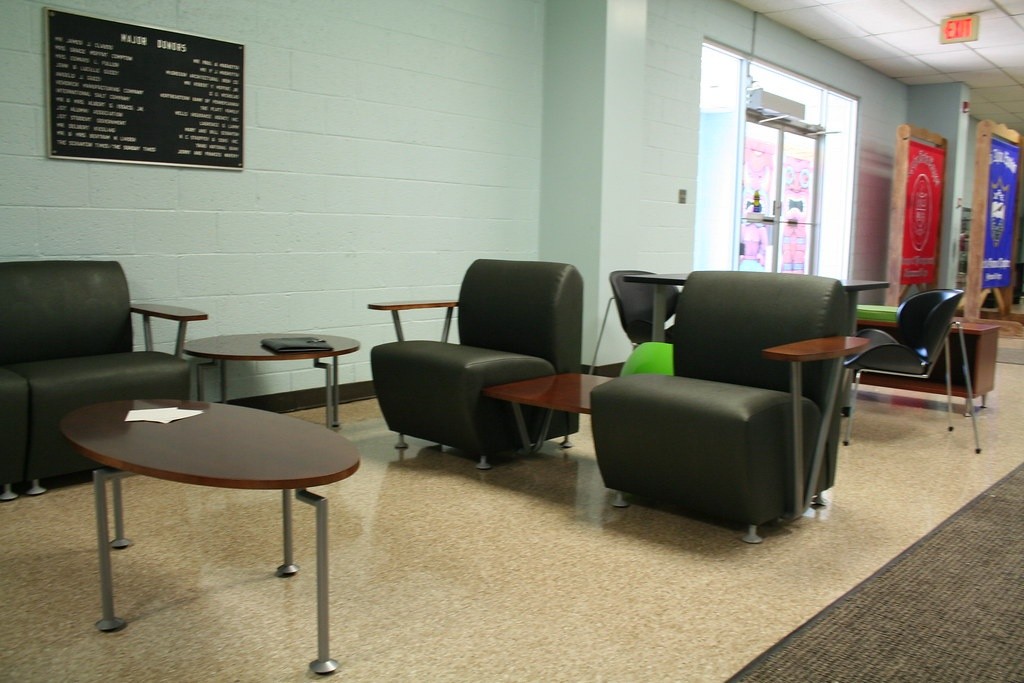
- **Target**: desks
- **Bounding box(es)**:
[839,278,889,336]
[59,400,362,674]
[180,334,361,427]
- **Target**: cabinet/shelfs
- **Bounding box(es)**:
[854,316,1001,415]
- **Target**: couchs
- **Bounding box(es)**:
[368,258,580,470]
[589,266,851,546]
[0,258,208,504]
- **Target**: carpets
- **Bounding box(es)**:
[723,463,1023,682]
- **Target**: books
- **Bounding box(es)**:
[260,337,334,353]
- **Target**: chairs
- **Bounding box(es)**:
[585,270,681,375]
[847,288,984,454]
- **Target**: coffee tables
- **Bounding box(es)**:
[480,373,616,458]
